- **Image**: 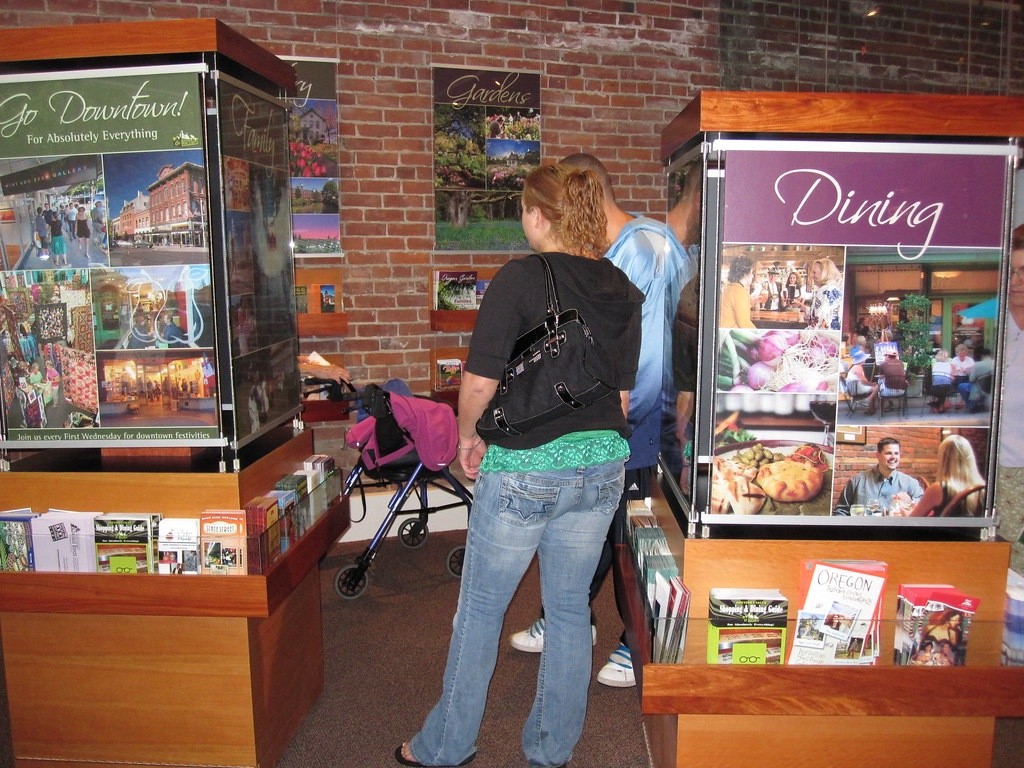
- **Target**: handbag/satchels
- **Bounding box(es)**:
[474,253,617,440]
[34,231,42,248]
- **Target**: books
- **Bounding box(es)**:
[264,455,337,549]
[158,518,201,574]
[707,560,982,666]
[0,507,103,573]
[201,496,284,575]
[625,500,690,664]
[93,510,163,573]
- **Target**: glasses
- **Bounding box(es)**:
[1010,268,1024,279]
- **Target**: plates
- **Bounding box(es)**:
[713,440,834,476]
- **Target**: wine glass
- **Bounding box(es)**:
[811,399,837,446]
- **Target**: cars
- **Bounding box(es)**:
[133,240,153,248]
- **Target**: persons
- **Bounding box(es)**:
[35,203,67,266]
[9,359,60,408]
[138,375,161,402]
[994,222,1024,541]
[20,323,37,362]
[322,290,334,312]
[248,367,294,435]
[294,183,303,199]
[68,200,108,260]
[160,311,182,348]
[297,354,350,385]
[910,607,963,665]
[232,295,257,357]
[126,315,152,349]
[393,156,666,767]
[800,613,859,658]
[489,108,540,138]
[719,253,985,518]
[163,376,189,406]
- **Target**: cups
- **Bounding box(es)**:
[849,504,865,516]
[789,284,795,303]
[866,497,879,512]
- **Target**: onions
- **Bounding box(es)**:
[727,328,841,393]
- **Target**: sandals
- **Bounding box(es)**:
[395,744,477,767]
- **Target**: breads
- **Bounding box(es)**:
[710,456,767,516]
[757,461,823,502]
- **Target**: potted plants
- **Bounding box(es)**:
[896,294,937,399]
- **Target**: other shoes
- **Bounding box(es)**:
[85,254,90,259]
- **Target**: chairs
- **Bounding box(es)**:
[915,475,988,518]
[839,356,994,421]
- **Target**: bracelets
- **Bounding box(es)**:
[458,439,483,450]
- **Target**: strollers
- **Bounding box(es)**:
[302,369,476,600]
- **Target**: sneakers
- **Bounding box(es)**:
[596,644,636,687]
[510,617,598,652]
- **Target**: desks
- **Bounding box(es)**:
[750,306,809,330]
